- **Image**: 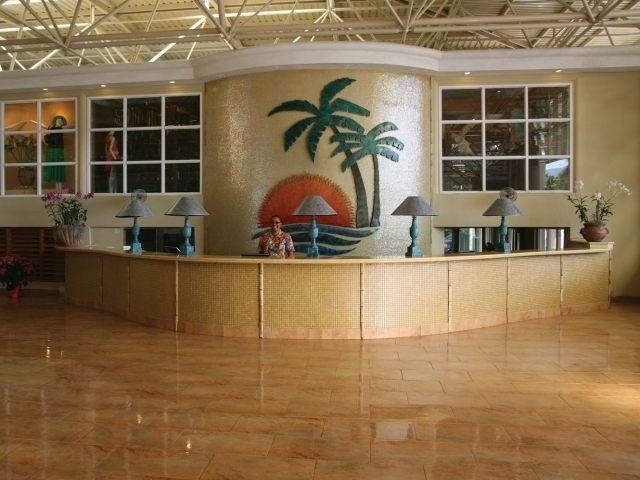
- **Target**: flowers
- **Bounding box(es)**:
[0,255,34,290]
[565,176,632,224]
[5,135,35,163]
[41,191,94,225]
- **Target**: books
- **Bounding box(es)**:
[455,228,566,253]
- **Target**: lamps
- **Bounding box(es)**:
[482,199,525,252]
[391,196,439,258]
[290,196,338,257]
[115,199,154,254]
[163,196,210,256]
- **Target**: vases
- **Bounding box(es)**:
[579,220,609,242]
[9,284,21,298]
[56,224,88,247]
[18,168,33,186]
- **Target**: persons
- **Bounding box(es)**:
[258,215,295,257]
[104,130,121,193]
[42,115,66,194]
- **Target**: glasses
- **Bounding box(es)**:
[271,221,281,224]
